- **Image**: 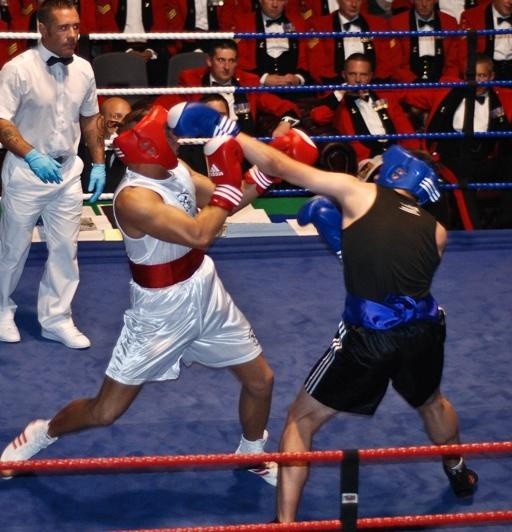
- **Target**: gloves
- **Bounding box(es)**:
[297,197,342,265]
[167,102,241,139]
[87,164,106,204]
[203,135,245,213]
[24,149,63,185]
[244,127,320,196]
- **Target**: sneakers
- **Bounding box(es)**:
[42,319,91,349]
[438,456,479,497]
[0,310,21,343]
[1,419,59,475]
[232,428,278,486]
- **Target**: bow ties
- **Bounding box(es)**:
[463,92,485,105]
[46,56,74,66]
[496,17,511,25]
[104,150,114,173]
[417,19,434,29]
[354,95,369,102]
[343,18,362,32]
[211,82,231,95]
[265,18,284,27]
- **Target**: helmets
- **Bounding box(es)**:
[378,145,443,206]
[111,106,179,170]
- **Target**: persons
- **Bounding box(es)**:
[389,1,460,159]
[0,104,319,485]
[0,0,106,350]
[311,1,404,84]
[78,97,131,193]
[174,94,250,177]
[179,1,229,53]
[154,39,302,141]
[166,101,477,524]
[428,55,512,199]
[312,52,473,230]
[95,0,187,63]
[229,0,311,88]
[461,0,512,122]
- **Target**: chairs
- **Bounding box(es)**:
[93,52,149,90]
[166,52,208,90]
